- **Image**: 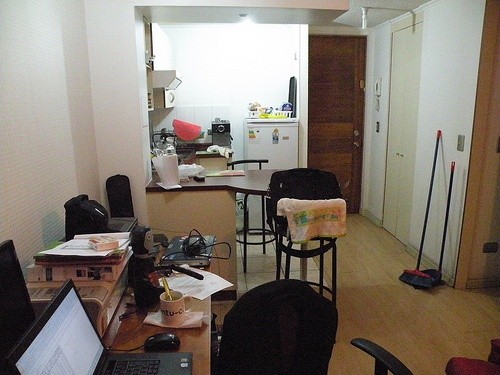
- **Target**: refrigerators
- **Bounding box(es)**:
[244,119,299,233]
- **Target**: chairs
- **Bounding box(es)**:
[210,279,415,375]
[266,168,347,306]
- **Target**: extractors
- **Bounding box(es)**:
[152,69,177,88]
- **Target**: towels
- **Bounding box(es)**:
[276,197,348,244]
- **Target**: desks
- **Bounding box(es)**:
[26,142,278,375]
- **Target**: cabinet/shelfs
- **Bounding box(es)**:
[144,19,176,112]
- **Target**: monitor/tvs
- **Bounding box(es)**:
[0,238,34,360]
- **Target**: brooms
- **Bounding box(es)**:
[399,129,441,289]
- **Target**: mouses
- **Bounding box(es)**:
[143,332,181,352]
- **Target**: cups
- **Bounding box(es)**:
[160,291,193,327]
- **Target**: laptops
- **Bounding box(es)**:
[158,235,215,266]
[2,277,193,375]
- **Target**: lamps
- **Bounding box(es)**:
[361,7,368,29]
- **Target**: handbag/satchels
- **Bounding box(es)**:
[152,150,180,186]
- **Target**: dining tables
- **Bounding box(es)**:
[227,160,278,273]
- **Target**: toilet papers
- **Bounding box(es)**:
[151,153,180,186]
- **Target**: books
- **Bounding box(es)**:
[24,240,129,283]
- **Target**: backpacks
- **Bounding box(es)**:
[63,194,108,241]
